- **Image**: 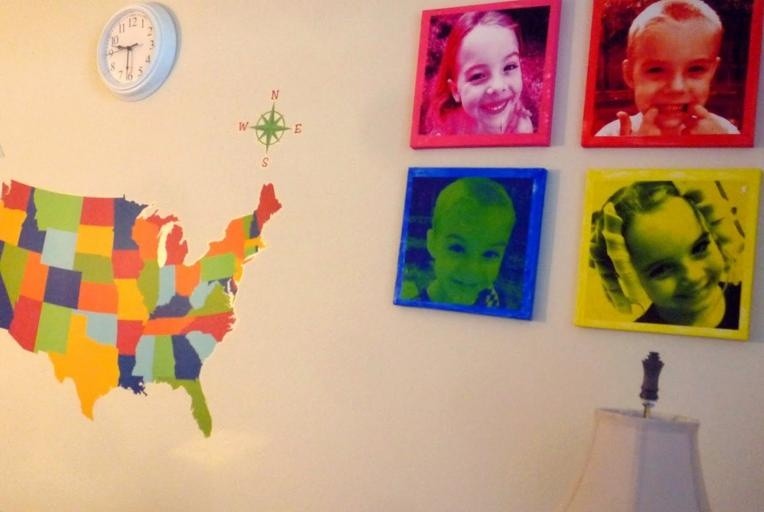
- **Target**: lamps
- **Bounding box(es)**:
[562,349,713,511]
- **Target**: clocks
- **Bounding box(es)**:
[95,3,177,104]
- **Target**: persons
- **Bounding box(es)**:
[409,177,523,311]
[595,1,743,136]
[425,11,534,135]
[587,181,744,330]
